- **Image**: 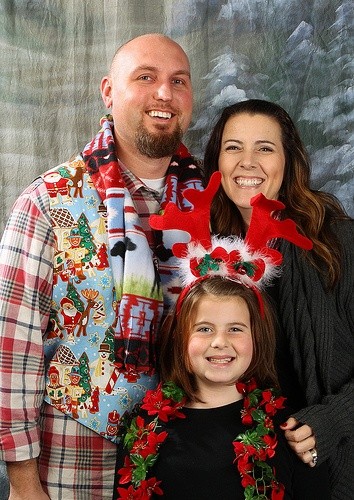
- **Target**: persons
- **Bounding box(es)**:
[0,33,211,500]
[203,99,354,500]
[112,171,312,500]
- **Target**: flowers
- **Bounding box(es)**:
[116,377,287,500]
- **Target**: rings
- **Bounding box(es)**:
[308,448,318,463]
